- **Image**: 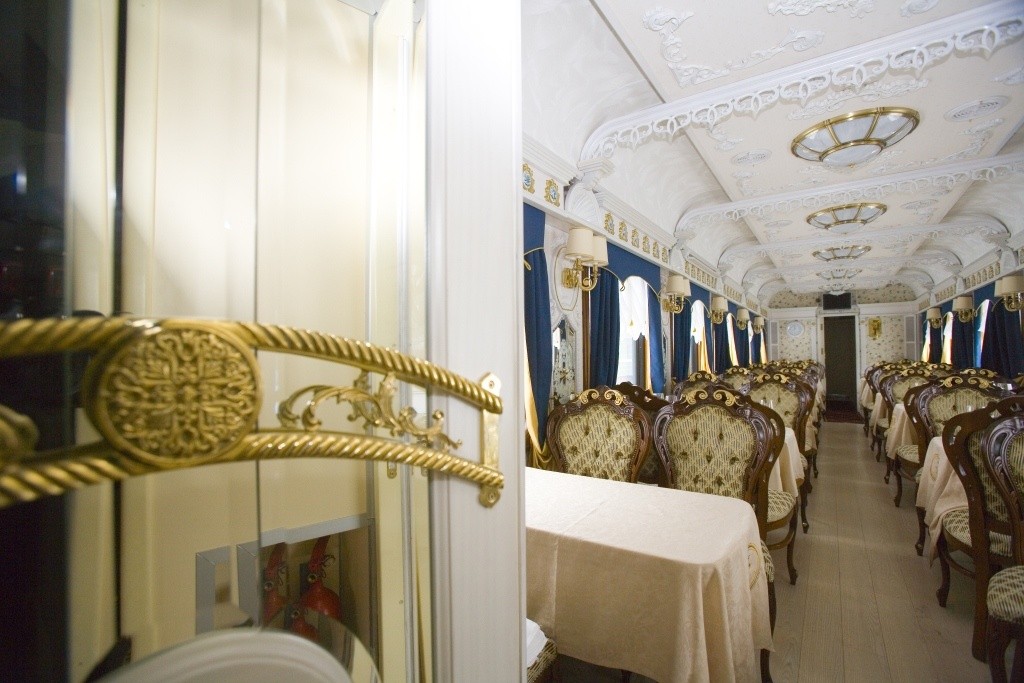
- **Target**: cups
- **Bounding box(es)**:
[997,383,1012,390]
[653,393,677,403]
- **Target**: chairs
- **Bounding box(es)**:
[524,356,1023,682]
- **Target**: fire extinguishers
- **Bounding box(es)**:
[263,543,286,628]
[295,535,339,641]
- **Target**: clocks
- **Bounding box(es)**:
[787,321,806,337]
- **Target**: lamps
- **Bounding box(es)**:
[557,231,608,293]
[925,276,1023,329]
[792,107,921,168]
[711,297,765,335]
[812,244,872,296]
[806,200,889,235]
[867,317,882,341]
[660,275,692,315]
